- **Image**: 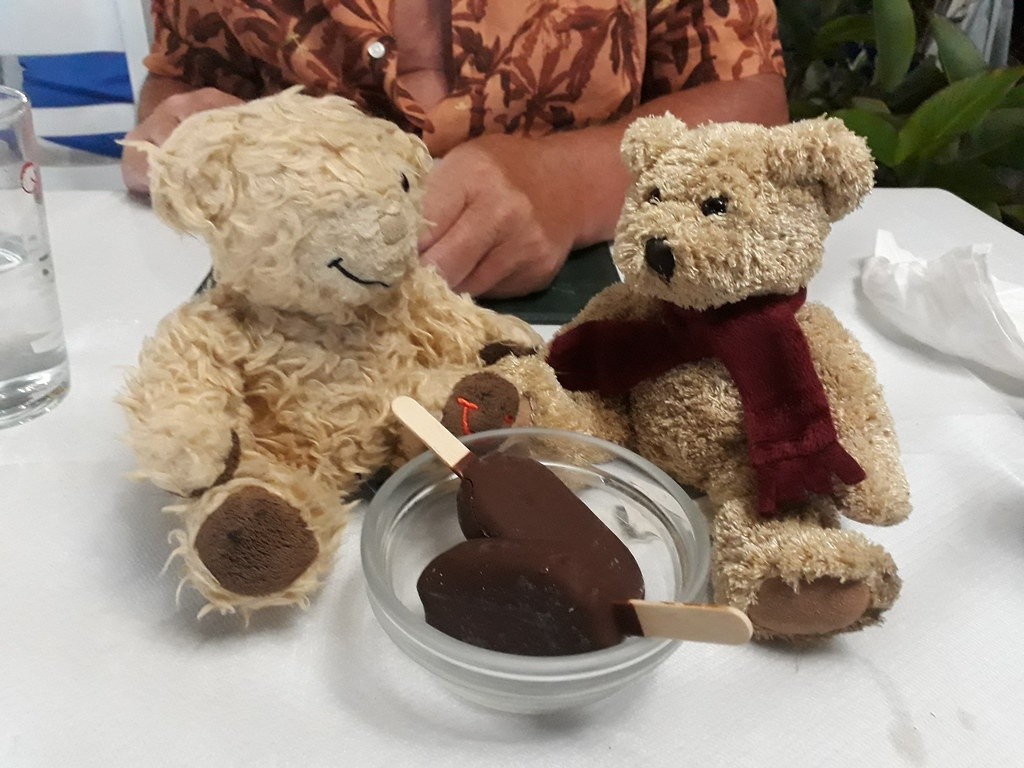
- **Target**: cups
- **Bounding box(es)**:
[0,84,69,427]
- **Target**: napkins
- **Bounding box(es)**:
[863,228,1024,383]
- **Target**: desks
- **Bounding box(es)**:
[0,189,1024,768]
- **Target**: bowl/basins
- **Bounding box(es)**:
[361,426,712,714]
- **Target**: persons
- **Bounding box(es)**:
[121,0,790,301]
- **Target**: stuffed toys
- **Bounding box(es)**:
[114,84,544,629]
[441,109,914,643]
